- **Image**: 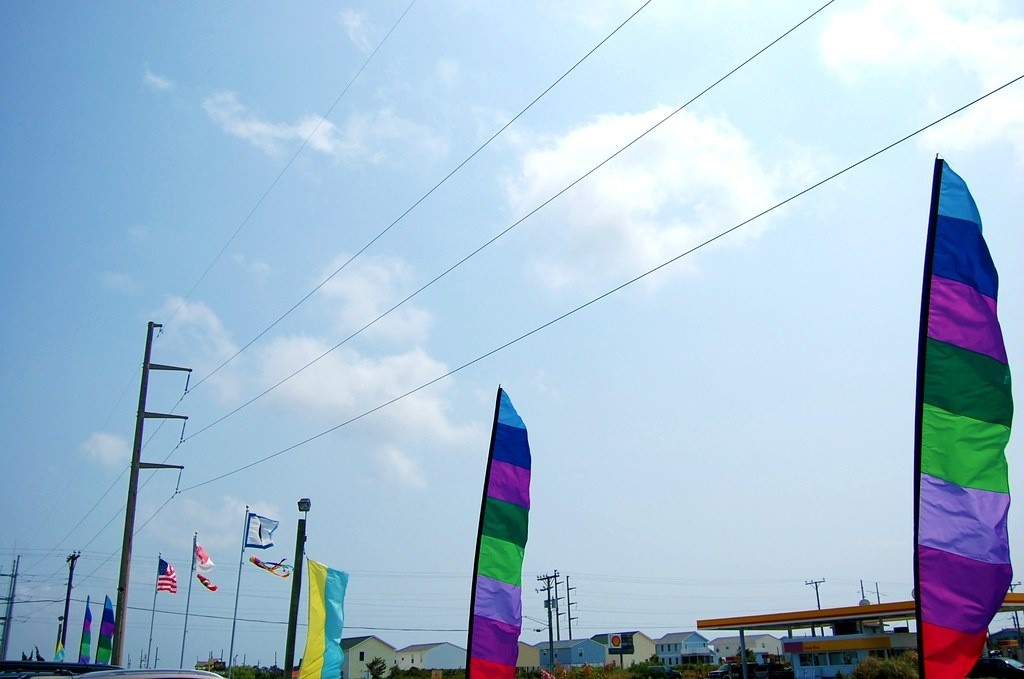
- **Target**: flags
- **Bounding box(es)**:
[244,513,279,548]
[299,560,348,679]
[156,559,177,594]
[194,546,214,572]
[54,640,66,662]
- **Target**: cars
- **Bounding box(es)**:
[646,666,683,679]
[756,663,795,679]
[967,657,1024,679]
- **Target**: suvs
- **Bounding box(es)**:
[708,663,755,679]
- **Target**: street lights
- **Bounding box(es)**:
[283,498,311,679]
[55,616,64,654]
